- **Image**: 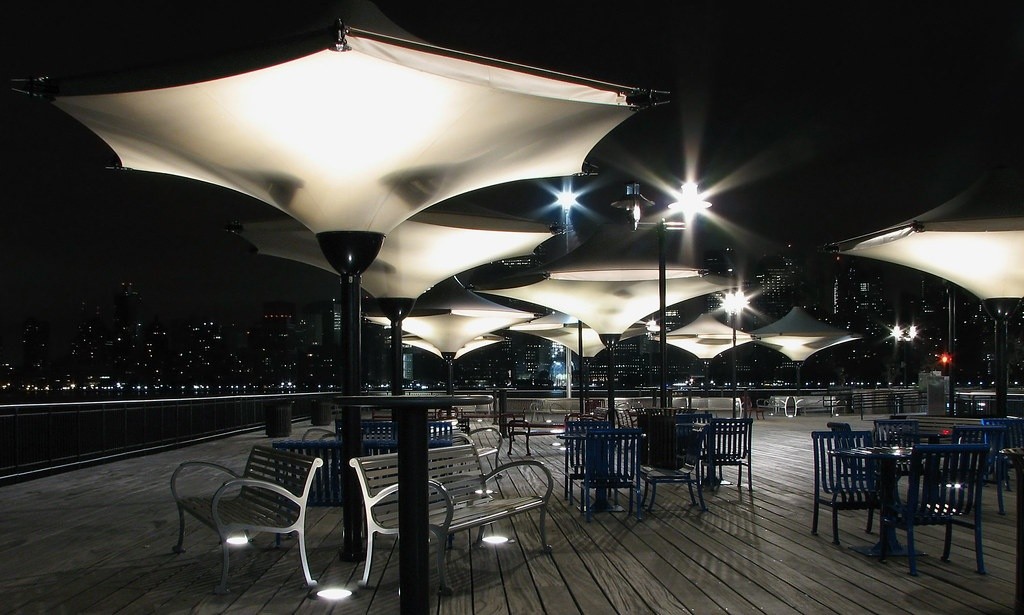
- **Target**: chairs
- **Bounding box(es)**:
[555,410,753,521]
[808,419,1024,576]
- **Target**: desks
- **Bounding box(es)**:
[832,445,931,560]
[556,433,647,503]
[676,422,741,485]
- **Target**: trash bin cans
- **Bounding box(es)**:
[887,393,905,414]
[308,396,334,427]
[634,406,699,472]
[835,393,853,413]
[997,448,1024,615]
[262,398,294,438]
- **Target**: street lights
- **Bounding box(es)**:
[722,288,747,420]
[892,324,917,389]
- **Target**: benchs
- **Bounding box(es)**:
[171,446,323,587]
[349,443,553,599]
[270,403,567,548]
[671,396,837,417]
